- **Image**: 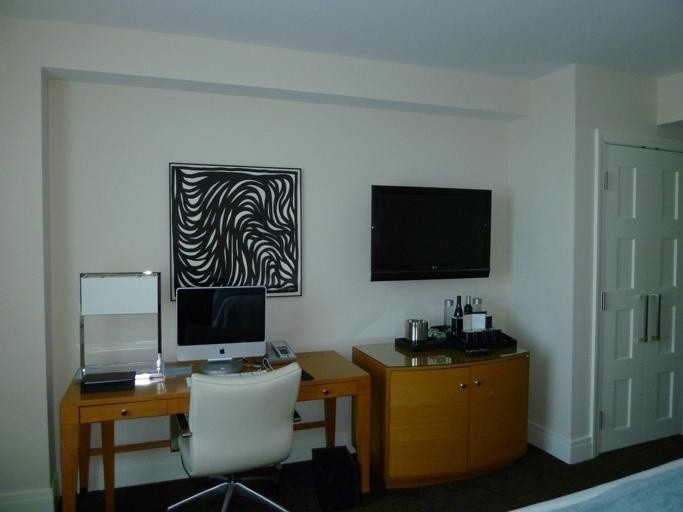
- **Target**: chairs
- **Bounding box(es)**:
[166,362,303,512]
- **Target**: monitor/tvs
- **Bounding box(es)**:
[175,286,266,374]
[370,184,492,282]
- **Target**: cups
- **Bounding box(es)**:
[404,319,427,342]
[405,356,428,367]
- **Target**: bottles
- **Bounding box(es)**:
[442,294,483,338]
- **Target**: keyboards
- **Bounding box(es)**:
[185,370,267,387]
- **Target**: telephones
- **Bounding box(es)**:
[265,340,298,363]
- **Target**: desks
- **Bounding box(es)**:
[59,350,372,512]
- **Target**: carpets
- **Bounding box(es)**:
[502,458,683,512]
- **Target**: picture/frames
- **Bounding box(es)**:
[169,162,303,301]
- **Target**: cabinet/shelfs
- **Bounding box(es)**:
[352,345,530,489]
